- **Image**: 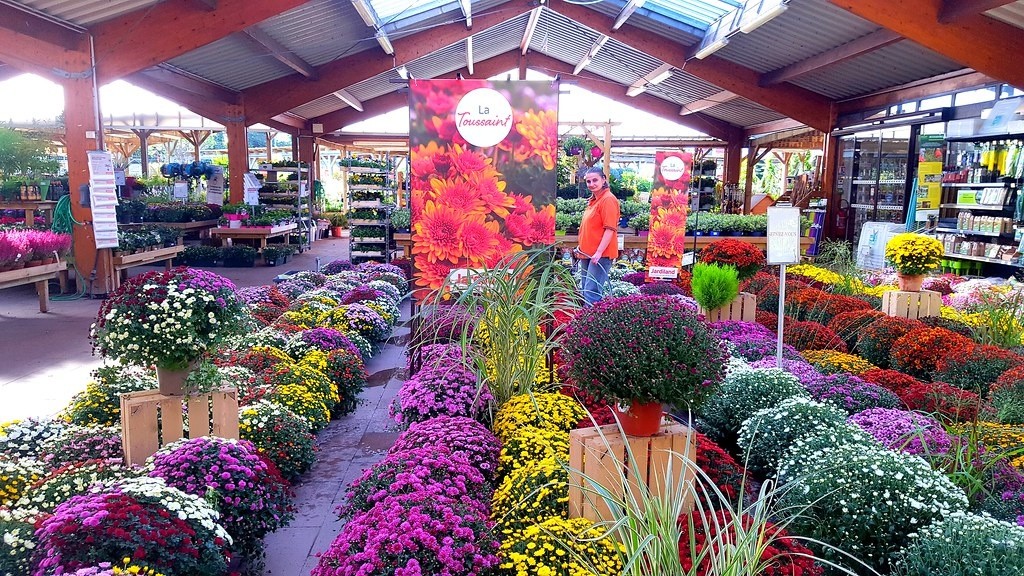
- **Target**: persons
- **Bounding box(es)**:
[575,166,621,311]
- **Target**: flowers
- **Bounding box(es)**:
[259,185,301,194]
[337,154,396,220]
[1,209,52,230]
[143,200,223,222]
[257,158,309,167]
[689,177,716,191]
[646,188,688,277]
[410,108,556,307]
[0,230,1024,576]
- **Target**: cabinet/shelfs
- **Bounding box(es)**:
[347,150,397,265]
[913,131,1024,280]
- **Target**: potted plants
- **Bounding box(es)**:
[104,137,164,219]
[563,137,602,157]
[690,260,756,323]
[112,221,188,256]
[329,212,348,237]
[0,125,70,201]
[186,200,308,265]
[552,155,812,235]
[390,207,412,232]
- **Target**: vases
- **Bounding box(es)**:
[339,165,381,173]
[0,257,63,271]
[610,397,667,436]
[350,198,390,208]
[258,192,310,197]
[258,167,308,172]
[153,359,197,393]
[348,185,384,191]
[899,271,928,293]
[348,218,392,225]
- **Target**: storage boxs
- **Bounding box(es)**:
[977,97,1024,134]
[920,122,947,138]
[947,118,984,136]
